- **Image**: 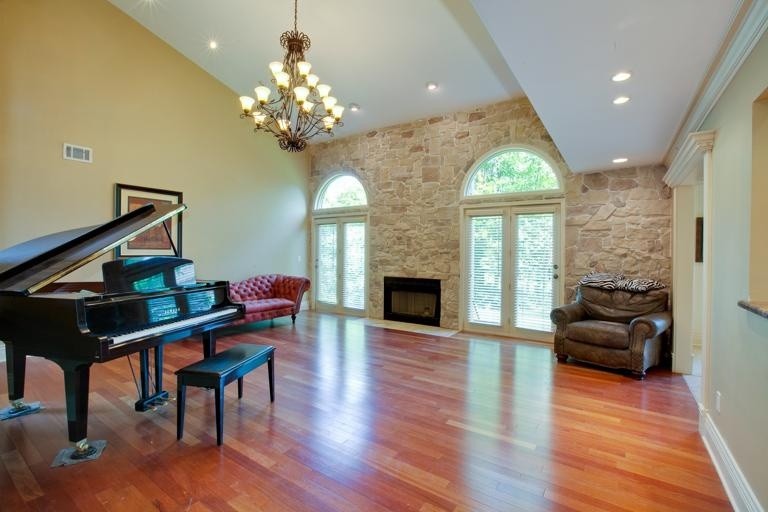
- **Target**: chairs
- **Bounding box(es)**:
[550,285,672,380]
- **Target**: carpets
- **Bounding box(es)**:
[353,316,460,338]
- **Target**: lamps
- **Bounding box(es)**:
[239,0,345,152]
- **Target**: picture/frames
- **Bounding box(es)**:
[114,183,183,261]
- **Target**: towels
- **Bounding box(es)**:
[49,440,106,467]
[0,401,41,421]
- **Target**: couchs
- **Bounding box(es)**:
[222,274,311,328]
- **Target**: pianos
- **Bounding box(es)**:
[0,202,247,458]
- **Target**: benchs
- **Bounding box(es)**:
[174,343,276,445]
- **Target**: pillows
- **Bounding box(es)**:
[575,273,666,292]
